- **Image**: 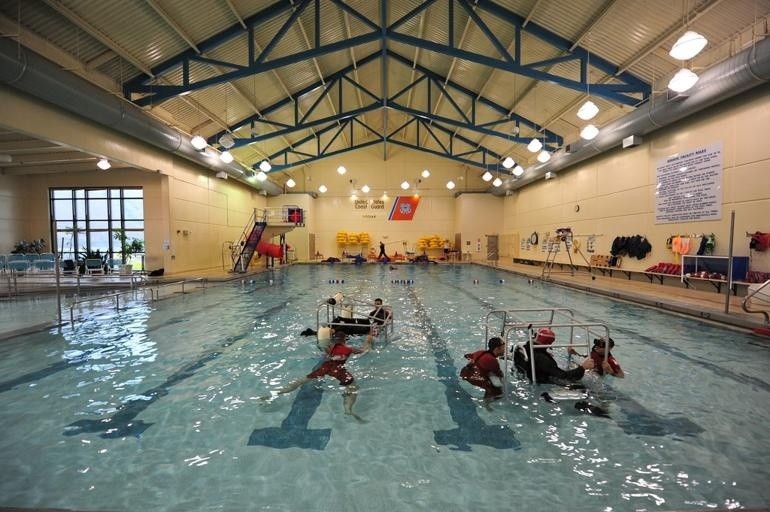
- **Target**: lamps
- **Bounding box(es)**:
[260,129,272,172]
[254,172,298,189]
[191,98,207,150]
[96,158,111,170]
[220,125,234,163]
[526,87,542,152]
[537,105,551,162]
[668,2,707,60]
[314,158,463,199]
[503,122,523,176]
[577,48,600,120]
[219,87,235,149]
[482,149,502,188]
[580,72,600,139]
[668,2,699,92]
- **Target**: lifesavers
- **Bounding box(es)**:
[336,231,369,245]
[369,247,377,254]
[429,255,437,259]
[416,235,455,250]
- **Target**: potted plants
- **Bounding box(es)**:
[113,228,143,275]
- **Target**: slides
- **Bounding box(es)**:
[248,226,292,258]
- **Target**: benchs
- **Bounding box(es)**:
[512,254,757,296]
[0,252,126,280]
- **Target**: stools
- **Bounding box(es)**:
[282,244,479,263]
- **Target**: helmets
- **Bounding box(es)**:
[535,328,555,345]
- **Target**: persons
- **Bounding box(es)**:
[377,241,389,260]
[461,338,504,412]
[514,328,594,384]
[368,298,392,325]
[260,331,372,415]
[568,337,624,378]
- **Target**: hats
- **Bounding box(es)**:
[332,331,345,344]
[594,336,615,348]
[488,337,505,348]
